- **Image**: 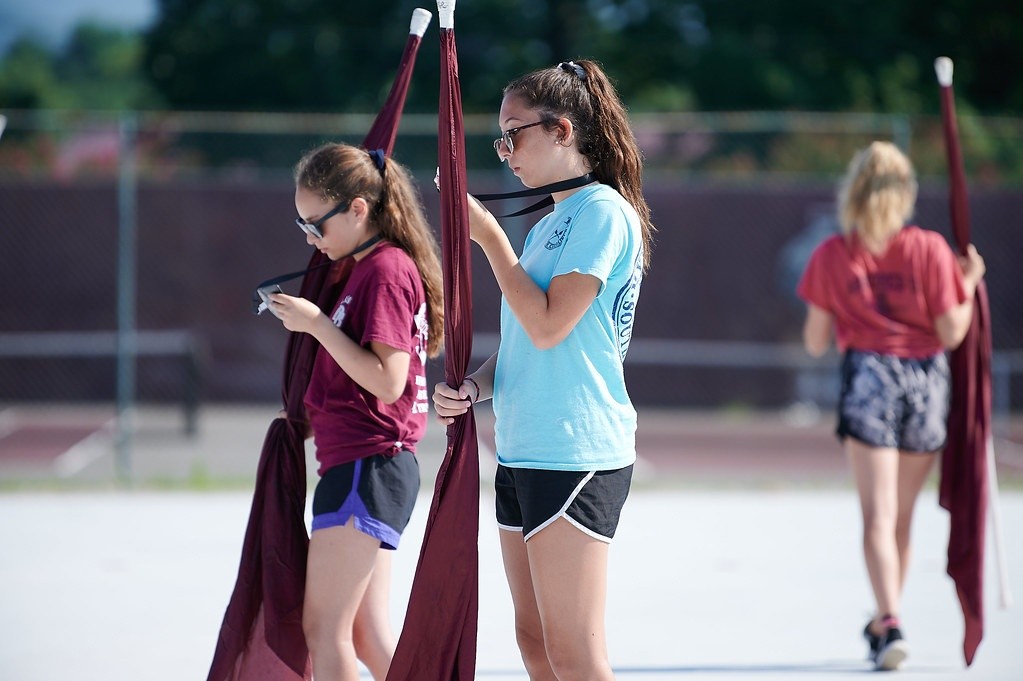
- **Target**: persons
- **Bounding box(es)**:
[800,140,986,671]
[433,60,657,681]
[265,143,445,681]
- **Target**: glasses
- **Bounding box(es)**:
[295,199,355,238]
[493,118,576,163]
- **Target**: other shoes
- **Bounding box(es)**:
[863,615,905,671]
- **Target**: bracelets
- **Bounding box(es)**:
[465,377,479,403]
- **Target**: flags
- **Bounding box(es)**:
[386,36,481,680]
[936,87,991,667]
[206,41,421,680]
